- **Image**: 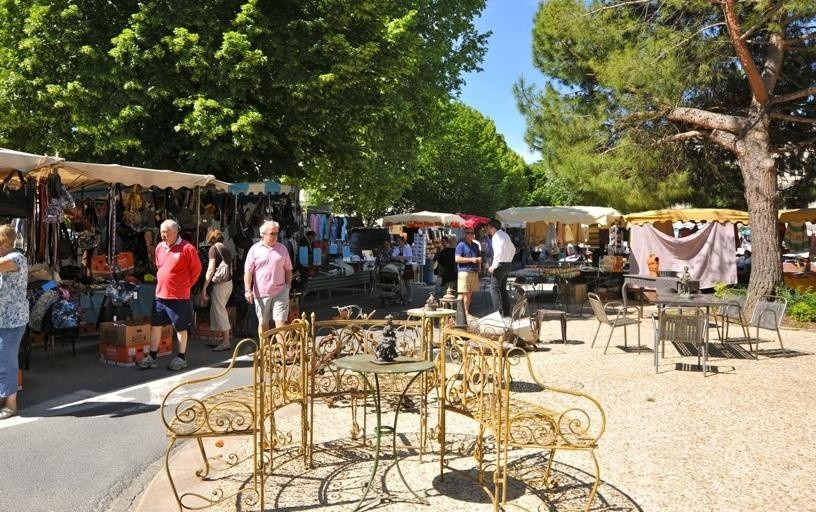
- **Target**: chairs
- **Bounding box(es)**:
[588,289,788,376]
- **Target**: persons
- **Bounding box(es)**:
[389,237,414,304]
[432,220,516,319]
[0,224,29,420]
[741,251,751,264]
[136,219,203,371]
[201,229,234,352]
[377,238,394,305]
[244,220,294,365]
[301,230,317,254]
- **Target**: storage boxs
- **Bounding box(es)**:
[90,251,134,282]
[557,283,587,303]
[98,315,174,368]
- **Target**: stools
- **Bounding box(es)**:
[46,334,76,363]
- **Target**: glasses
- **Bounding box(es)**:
[263,232,277,235]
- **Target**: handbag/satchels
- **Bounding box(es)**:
[177,209,212,231]
[117,209,142,240]
[228,216,258,249]
[43,174,76,223]
[212,260,229,283]
[0,183,31,218]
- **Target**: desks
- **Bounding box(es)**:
[304,271,370,303]
[622,274,656,314]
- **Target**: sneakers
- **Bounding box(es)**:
[205,341,230,351]
[167,357,187,370]
[136,355,157,368]
[0,407,16,419]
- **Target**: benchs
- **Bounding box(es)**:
[162,315,306,511]
[257,315,444,469]
[436,327,606,510]
